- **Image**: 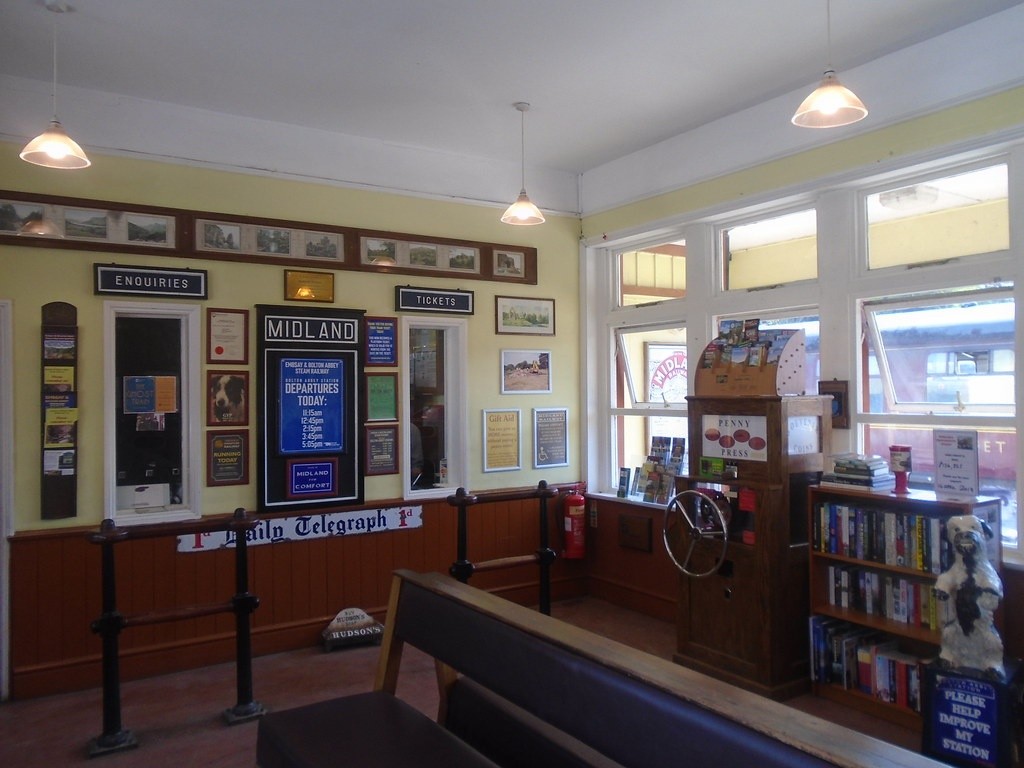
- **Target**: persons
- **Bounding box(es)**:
[410,423,424,485]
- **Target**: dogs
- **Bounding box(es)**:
[932,515,1008,685]
[212,375,246,422]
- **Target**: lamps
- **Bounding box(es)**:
[791,0,867,128]
[19,0,92,170]
[501,101,546,225]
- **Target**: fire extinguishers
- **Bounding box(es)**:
[563,485,587,558]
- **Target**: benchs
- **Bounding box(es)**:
[257,568,953,768]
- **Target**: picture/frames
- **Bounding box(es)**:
[206,370,250,426]
[501,348,553,394]
[0,186,541,285]
[818,380,850,429]
[495,294,555,337]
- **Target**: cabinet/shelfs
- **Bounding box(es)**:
[807,480,1003,731]
[673,393,834,703]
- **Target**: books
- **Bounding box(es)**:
[821,456,895,491]
[808,500,954,713]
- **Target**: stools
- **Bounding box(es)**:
[919,654,1024,768]
